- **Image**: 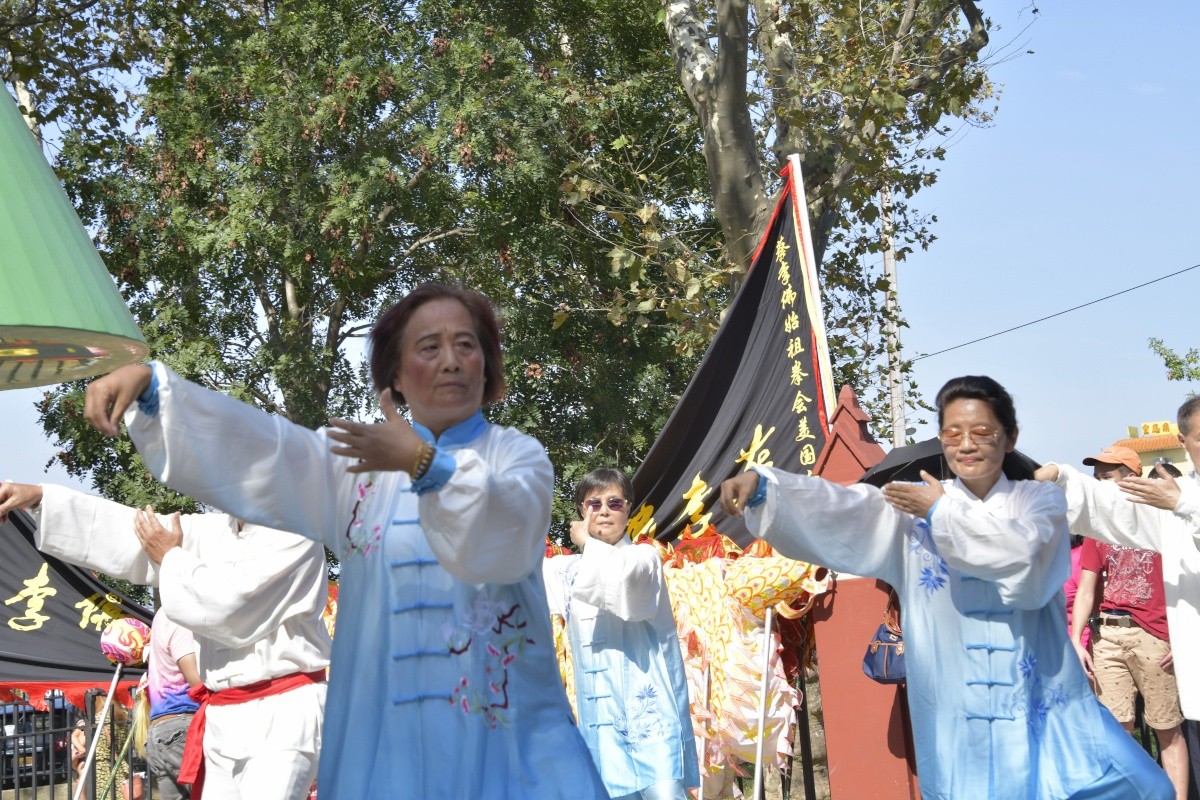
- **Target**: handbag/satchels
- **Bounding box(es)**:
[863,618,912,686]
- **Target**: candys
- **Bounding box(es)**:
[100,617,153,667]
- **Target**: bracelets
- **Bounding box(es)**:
[579,543,585,555]
[411,440,434,481]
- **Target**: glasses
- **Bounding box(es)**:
[939,427,1000,445]
[582,497,626,512]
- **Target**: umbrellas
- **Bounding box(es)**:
[857,436,1043,488]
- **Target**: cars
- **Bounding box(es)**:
[0,715,79,790]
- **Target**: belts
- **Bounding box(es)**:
[155,713,195,723]
[1097,617,1144,628]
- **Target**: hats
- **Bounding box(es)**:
[1148,456,1184,477]
[1083,447,1144,477]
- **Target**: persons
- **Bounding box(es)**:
[542,467,701,800]
[71,719,86,800]
[719,376,1199,800]
[84,280,610,799]
[0,481,329,800]
